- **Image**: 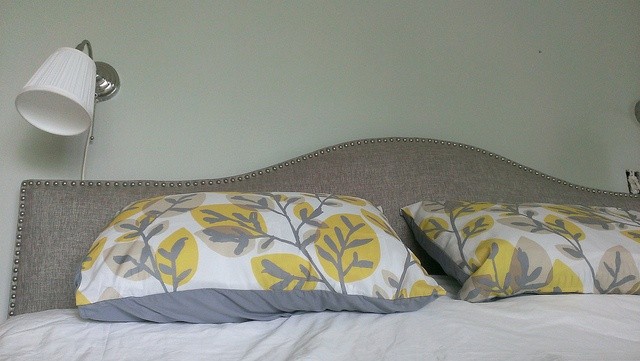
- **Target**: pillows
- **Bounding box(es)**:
[75,191,447,324]
[399,199,640,304]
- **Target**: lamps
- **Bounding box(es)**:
[14,41,97,181]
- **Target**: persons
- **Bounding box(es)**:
[628,171,640,196]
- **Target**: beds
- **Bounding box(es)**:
[0,138,640,361]
